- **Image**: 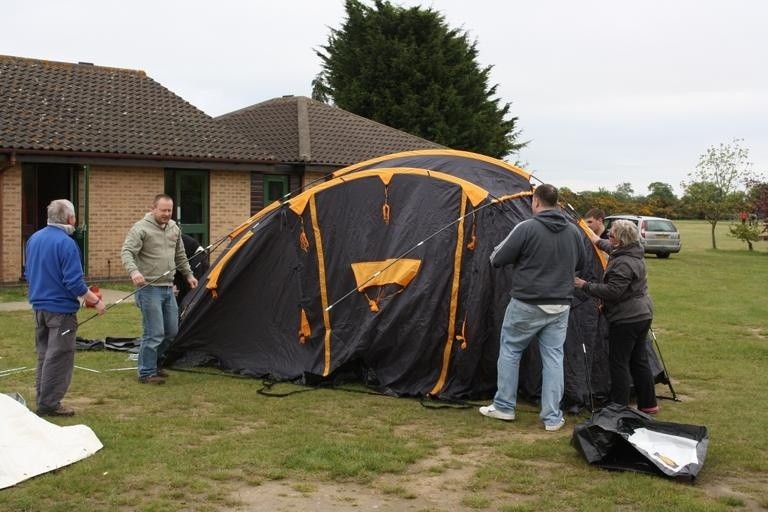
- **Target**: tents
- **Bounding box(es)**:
[124,142,680,413]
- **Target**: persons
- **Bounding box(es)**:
[166,230,210,303]
[740,210,748,224]
[571,219,661,413]
[582,207,613,243]
[119,193,199,386]
[25,198,107,417]
[477,183,590,432]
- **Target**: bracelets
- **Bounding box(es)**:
[92,298,100,306]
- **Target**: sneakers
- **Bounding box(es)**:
[157,368,169,378]
[478,403,516,421]
[138,373,166,386]
[545,415,566,432]
[35,401,74,418]
[638,406,659,414]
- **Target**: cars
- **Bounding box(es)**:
[602,215,681,258]
